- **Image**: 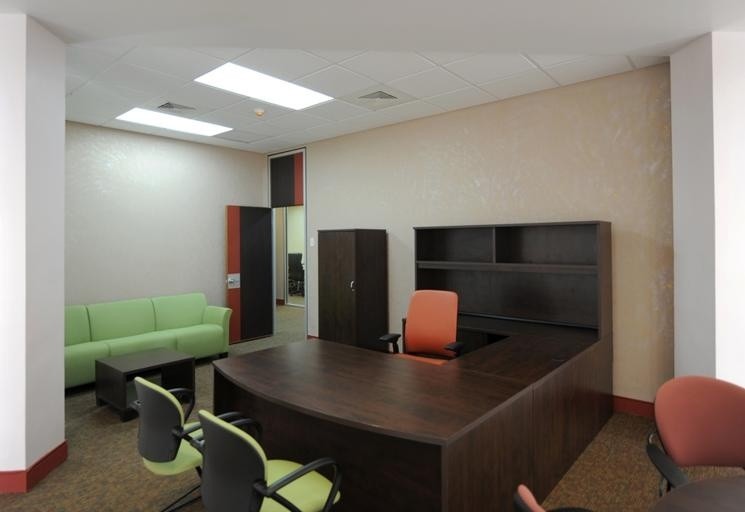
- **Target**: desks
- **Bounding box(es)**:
[211,339,613,512]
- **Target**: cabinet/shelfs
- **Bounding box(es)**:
[318,229,388,351]
[413,219,613,339]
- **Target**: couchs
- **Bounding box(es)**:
[65,292,233,396]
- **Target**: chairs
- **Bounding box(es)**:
[379,290,465,366]
[198,410,342,512]
[288,253,304,297]
[514,484,593,512]
[646,375,745,496]
[130,376,250,477]
